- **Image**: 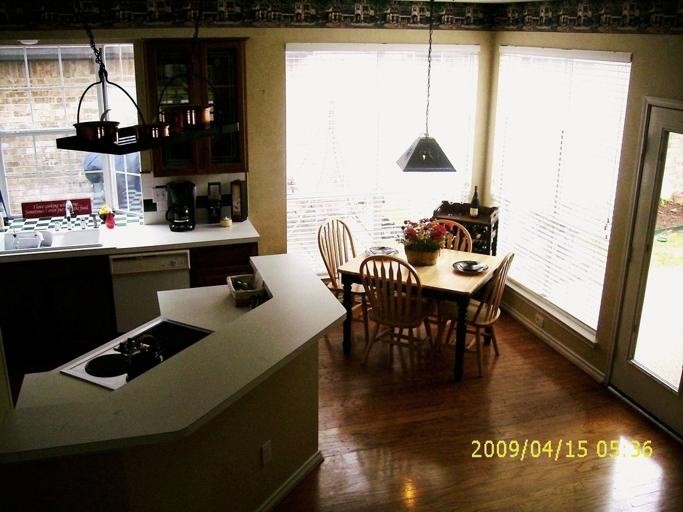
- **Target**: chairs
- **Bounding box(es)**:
[315,219,382,345]
[431,252,515,376]
[433,220,473,252]
[360,257,436,378]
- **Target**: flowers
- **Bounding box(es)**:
[399,217,454,249]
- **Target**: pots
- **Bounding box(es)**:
[75,109,119,142]
[165,105,211,131]
[133,104,170,140]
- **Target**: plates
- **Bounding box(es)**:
[453,259,489,276]
[364,246,399,260]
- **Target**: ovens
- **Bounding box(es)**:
[111,251,190,333]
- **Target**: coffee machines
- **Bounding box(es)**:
[165,181,196,232]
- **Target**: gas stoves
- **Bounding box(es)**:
[60,318,214,390]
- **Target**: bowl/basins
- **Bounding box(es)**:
[221,219,233,227]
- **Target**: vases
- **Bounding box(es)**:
[404,244,439,266]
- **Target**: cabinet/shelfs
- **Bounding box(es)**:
[133,35,250,179]
[429,200,499,304]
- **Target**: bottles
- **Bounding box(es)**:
[470,186,480,219]
[65,199,75,218]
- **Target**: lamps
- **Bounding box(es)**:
[54,20,238,155]
[395,3,458,172]
